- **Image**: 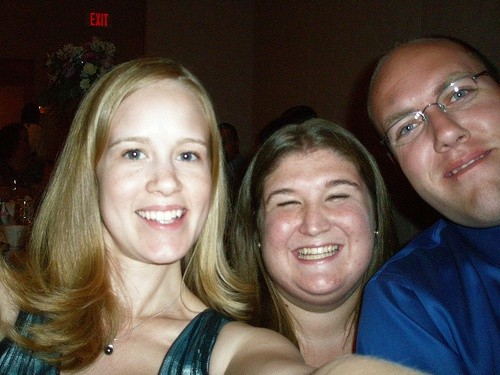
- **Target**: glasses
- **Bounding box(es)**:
[380,70,484,150]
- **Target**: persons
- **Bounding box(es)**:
[0,105,47,203]
[227,118,389,368]
[0,58,432,375]
[354,35,500,375]
[218,106,318,210]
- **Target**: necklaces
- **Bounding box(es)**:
[104,288,185,355]
